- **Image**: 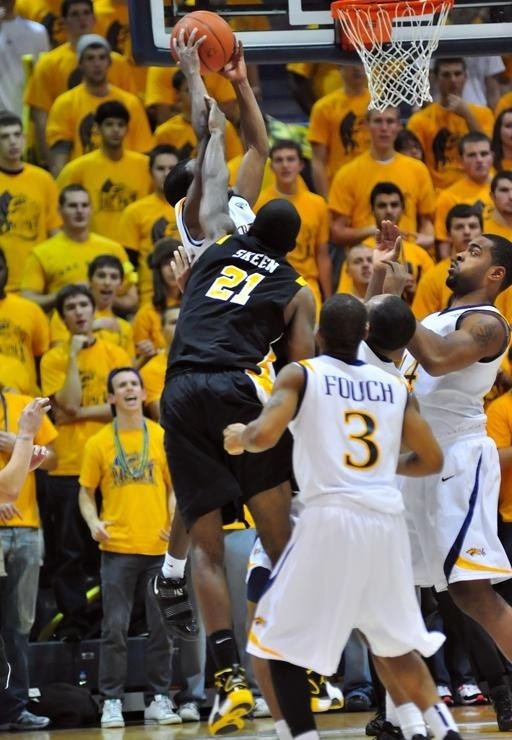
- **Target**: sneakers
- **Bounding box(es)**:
[1,707,50,731]
[149,570,200,641]
[100,683,512,739]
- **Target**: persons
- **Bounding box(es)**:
[0,0,512,740]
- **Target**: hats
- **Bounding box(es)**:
[152,239,183,266]
[77,34,112,63]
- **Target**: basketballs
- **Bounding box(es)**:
[171,11,235,72]
